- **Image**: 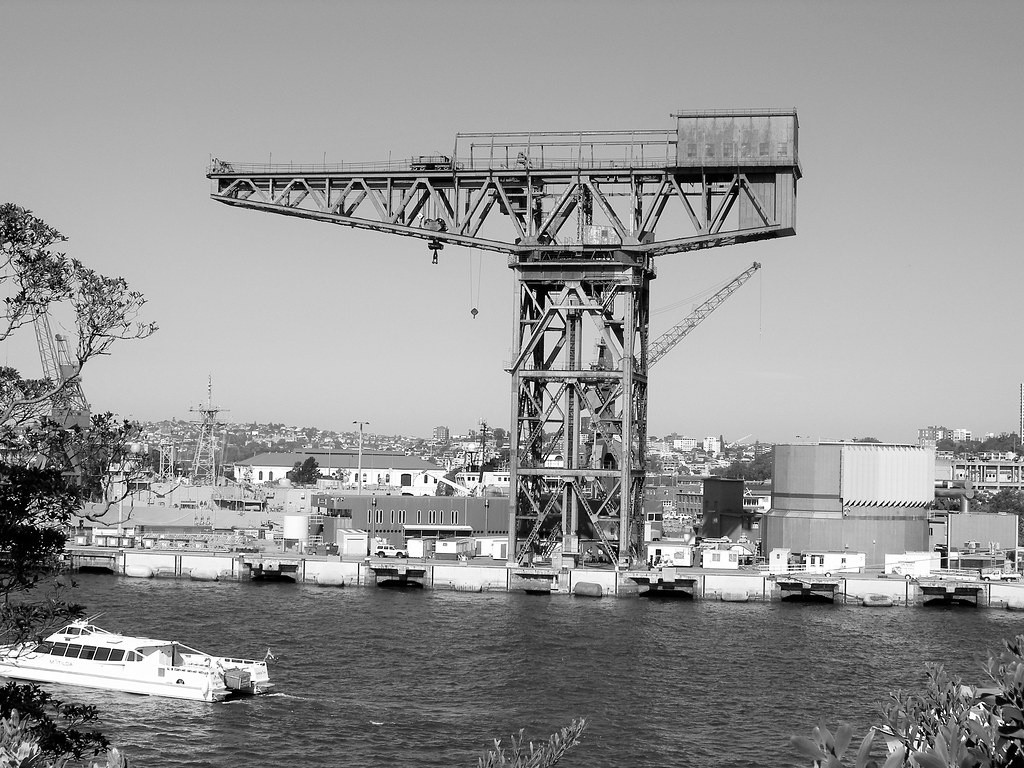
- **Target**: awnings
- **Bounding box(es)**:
[402,524,474,548]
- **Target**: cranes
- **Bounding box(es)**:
[584,259,763,476]
[207,108,806,576]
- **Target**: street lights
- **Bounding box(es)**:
[352,420,369,495]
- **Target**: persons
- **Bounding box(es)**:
[587,546,593,562]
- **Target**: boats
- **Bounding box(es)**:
[0,619,275,704]
